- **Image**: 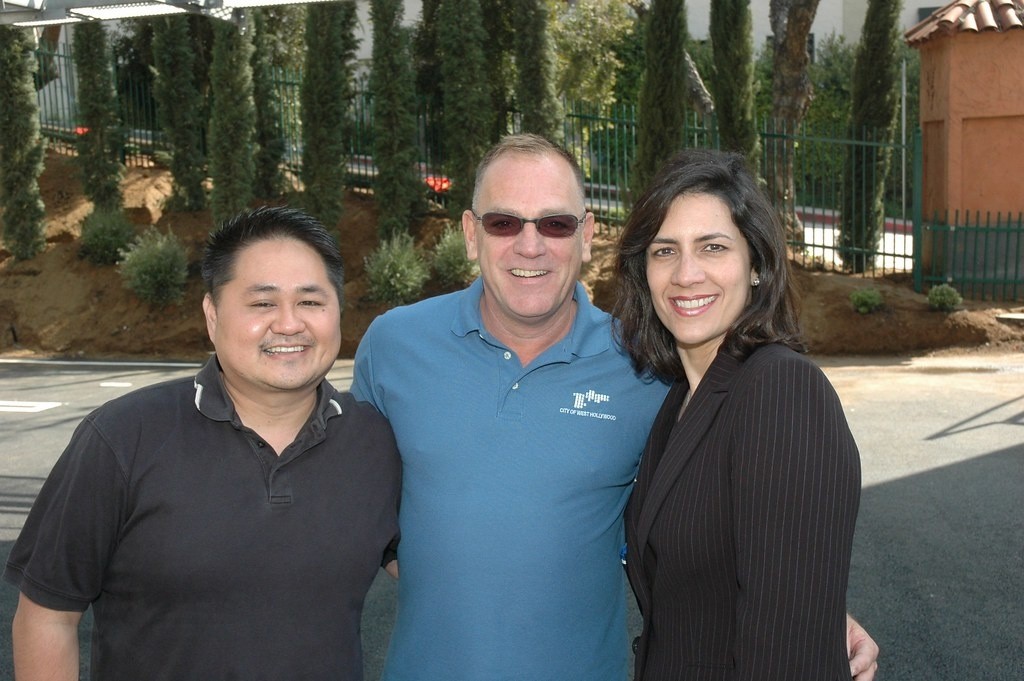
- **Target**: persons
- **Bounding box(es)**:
[349,134,880,681]
[609,149,862,681]
[2,207,403,681]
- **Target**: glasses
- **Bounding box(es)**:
[476,211,583,238]
